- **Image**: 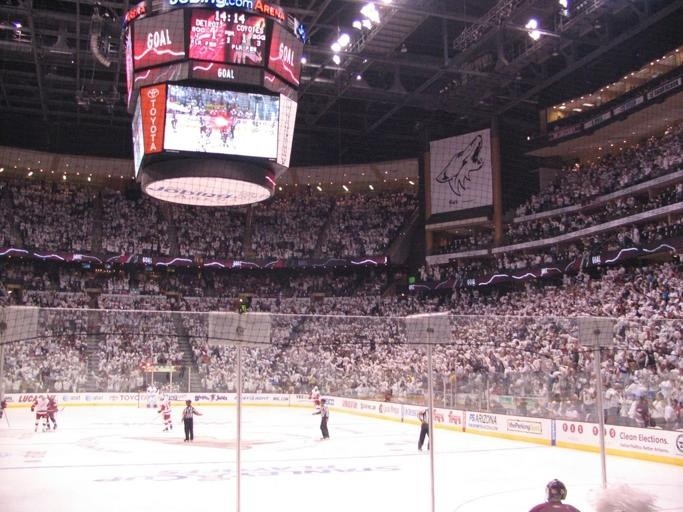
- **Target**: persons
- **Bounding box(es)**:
[169,85,255,147]
[182,400,200,442]
[417,407,430,451]
[313,399,329,440]
[0,125,682,431]
[531,479,579,512]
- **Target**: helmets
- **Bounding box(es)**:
[544,479,568,506]
